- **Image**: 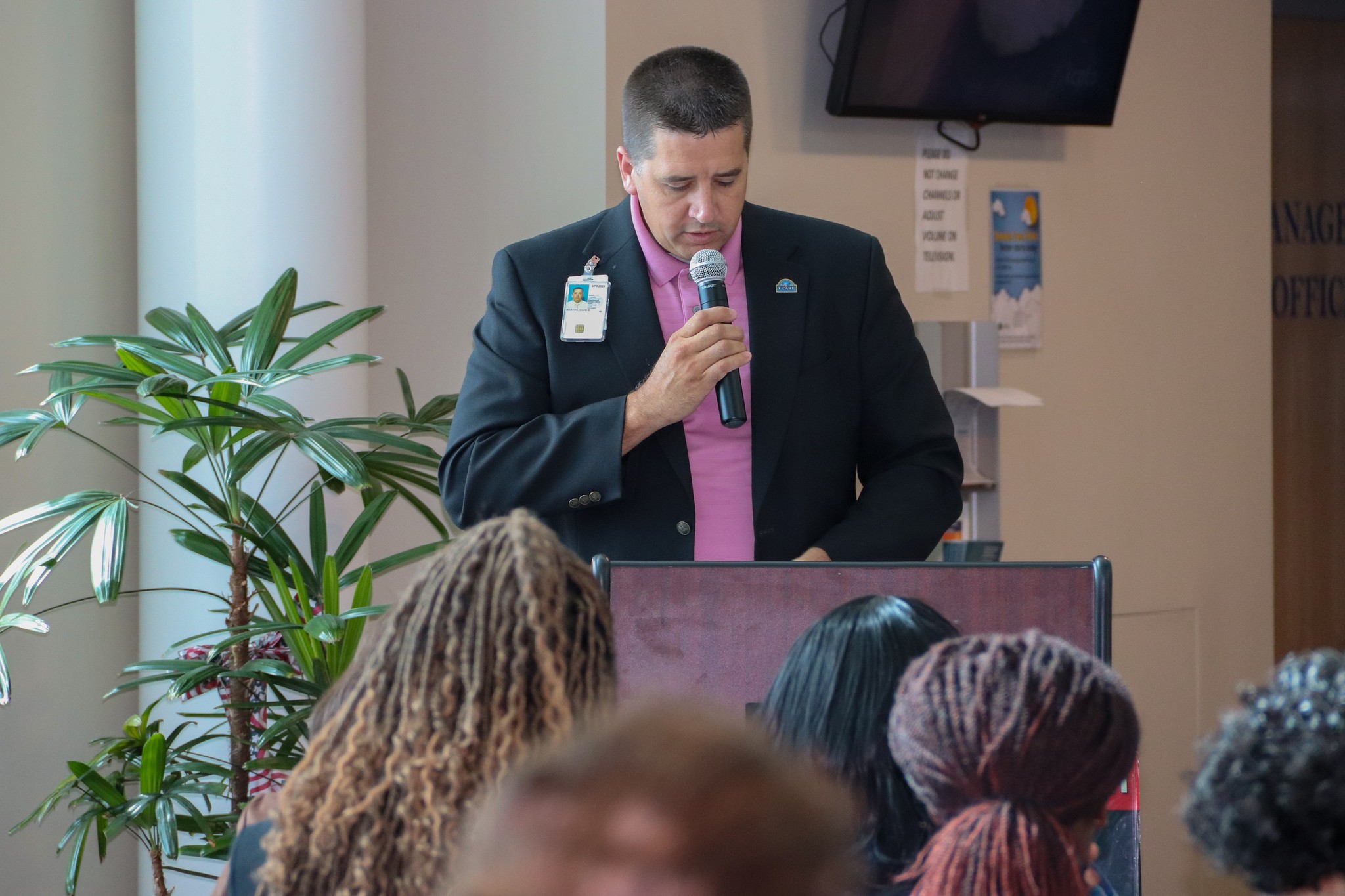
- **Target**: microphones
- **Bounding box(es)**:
[689,249,747,430]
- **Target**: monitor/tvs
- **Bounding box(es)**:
[826,0,1141,129]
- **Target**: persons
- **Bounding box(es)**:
[1183,647,1345,896]
[743,592,966,896]
[438,684,870,896]
[440,45,966,565]
[886,623,1143,896]
[221,509,620,896]
